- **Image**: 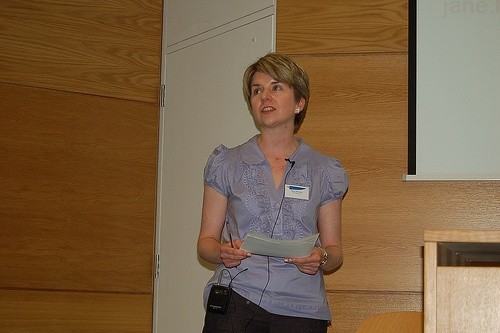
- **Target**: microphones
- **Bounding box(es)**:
[291,161,295,167]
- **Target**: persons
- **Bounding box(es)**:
[197,52,349,333]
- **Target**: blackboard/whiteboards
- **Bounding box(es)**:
[402,0,500,181]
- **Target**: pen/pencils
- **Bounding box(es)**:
[229,233,238,268]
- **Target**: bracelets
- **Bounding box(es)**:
[321,251,328,266]
